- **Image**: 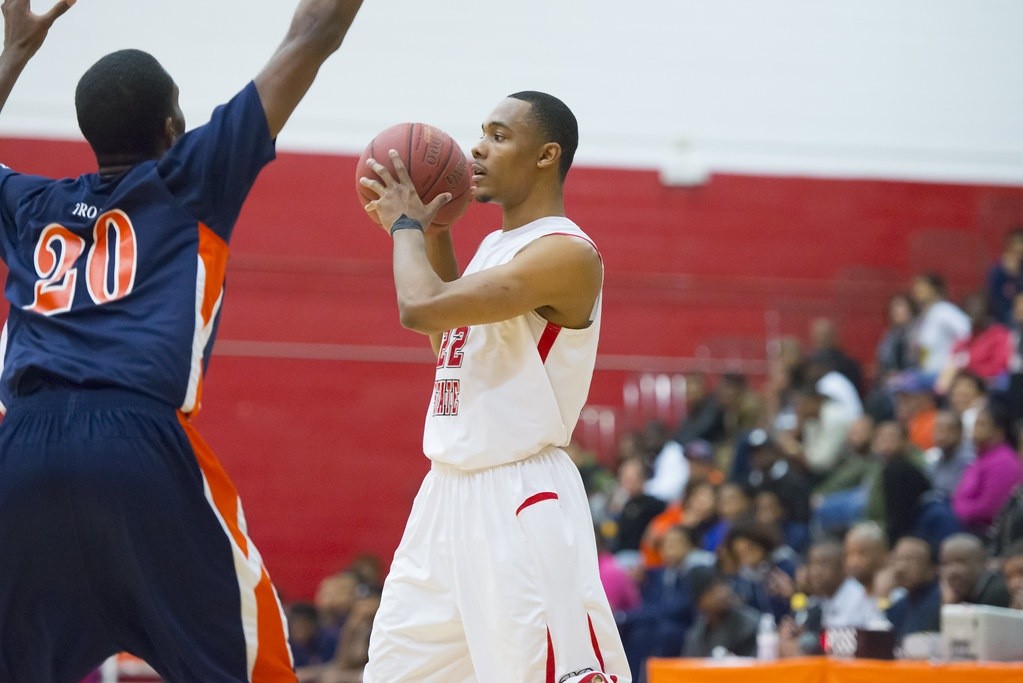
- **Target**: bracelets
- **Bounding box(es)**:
[390,213,424,235]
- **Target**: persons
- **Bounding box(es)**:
[359,91,634,683]
[0,0,363,683]
[566,223,1023,683]
[282,553,384,683]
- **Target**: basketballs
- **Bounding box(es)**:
[355,123,474,231]
